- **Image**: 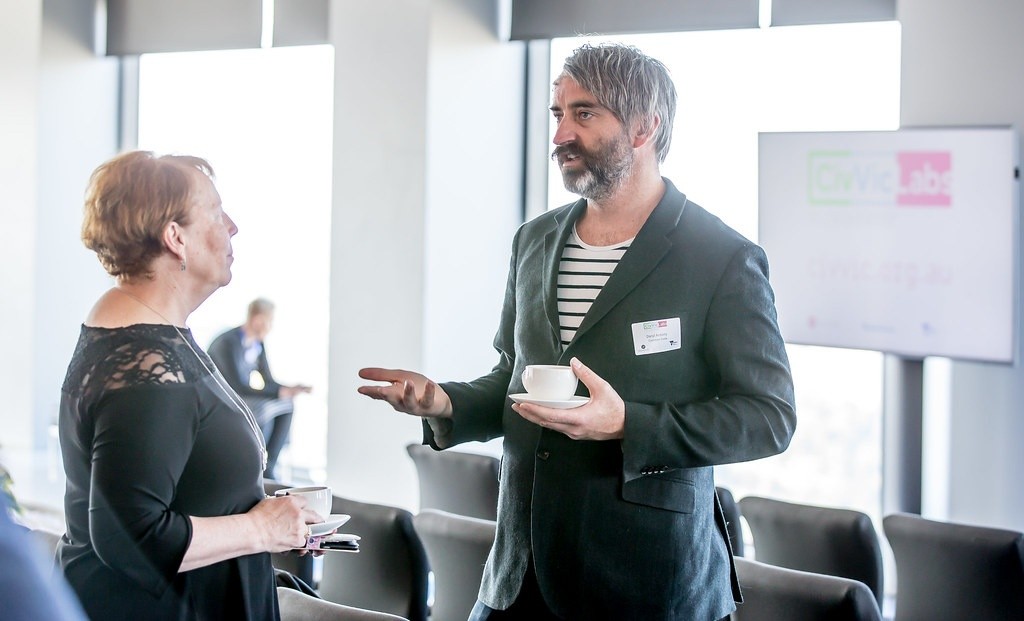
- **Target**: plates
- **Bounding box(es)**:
[508,391,590,409]
[307,513,351,536]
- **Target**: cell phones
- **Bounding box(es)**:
[319,540,360,550]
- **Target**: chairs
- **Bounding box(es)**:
[733,554,881,621]
[406,443,500,522]
[882,512,1024,621]
[739,496,884,613]
[320,495,429,621]
[411,508,497,621]
[277,586,409,621]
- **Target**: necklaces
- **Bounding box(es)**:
[112,285,268,471]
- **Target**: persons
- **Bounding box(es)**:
[55,152,338,621]
[357,43,797,621]
[207,297,314,482]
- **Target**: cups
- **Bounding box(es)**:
[275,486,333,524]
[522,364,579,400]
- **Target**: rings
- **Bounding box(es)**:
[305,525,310,538]
